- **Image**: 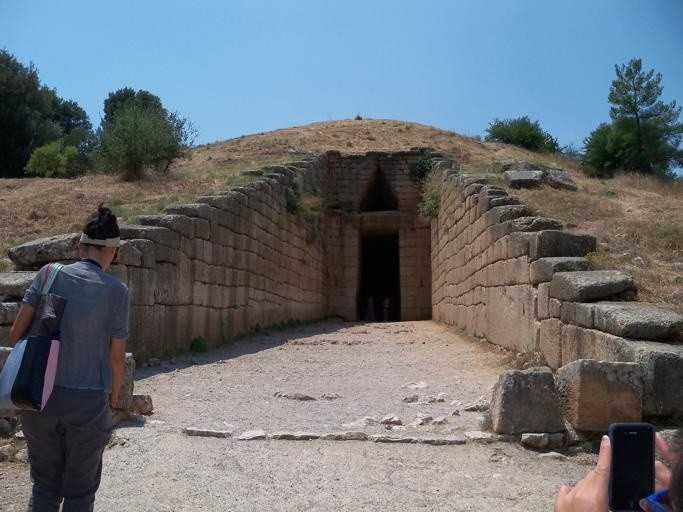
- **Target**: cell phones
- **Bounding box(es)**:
[608,422,655,512]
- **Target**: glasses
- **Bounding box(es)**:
[646,489,672,512]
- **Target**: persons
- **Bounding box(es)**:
[9,201,130,512]
[553,422,682,512]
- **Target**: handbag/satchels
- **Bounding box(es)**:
[0,294,66,411]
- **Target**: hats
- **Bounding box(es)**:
[80,232,120,261]
